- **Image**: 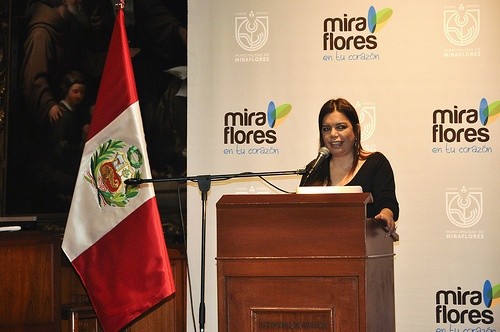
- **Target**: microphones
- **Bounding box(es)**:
[308,146,330,177]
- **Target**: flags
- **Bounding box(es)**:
[60,0,176,332]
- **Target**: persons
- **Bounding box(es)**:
[297,98,401,239]
